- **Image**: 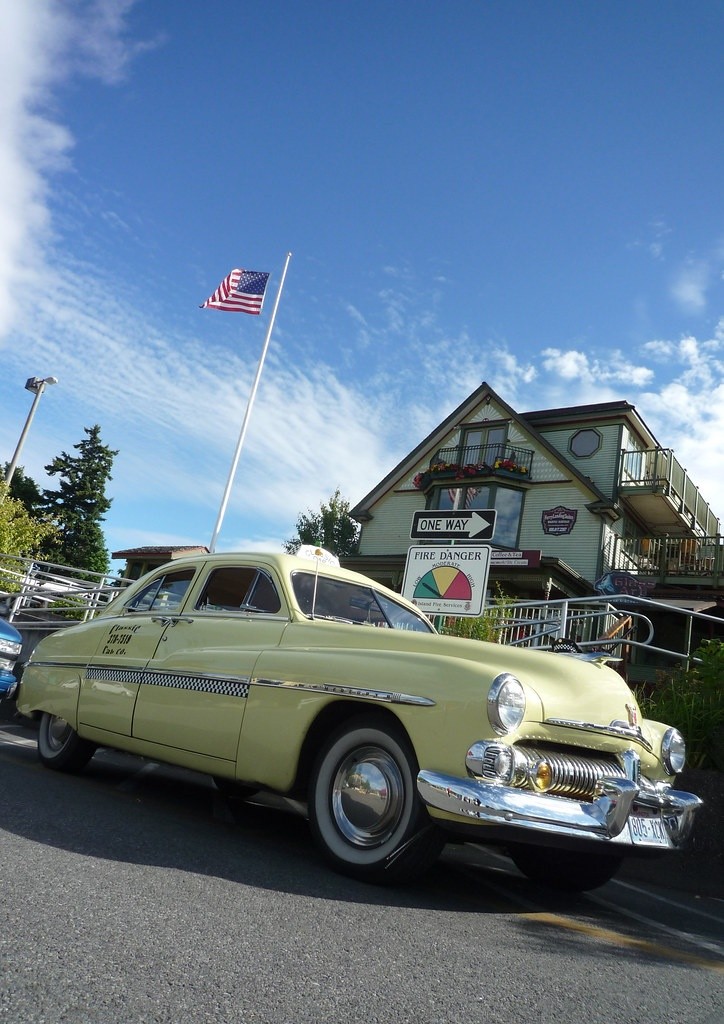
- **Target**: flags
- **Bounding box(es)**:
[199,266,271,315]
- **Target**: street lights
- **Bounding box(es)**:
[1,376,58,505]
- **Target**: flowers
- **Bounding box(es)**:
[412,458,527,488]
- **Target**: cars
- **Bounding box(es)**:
[0,544,703,894]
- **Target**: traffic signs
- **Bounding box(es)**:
[410,509,498,540]
